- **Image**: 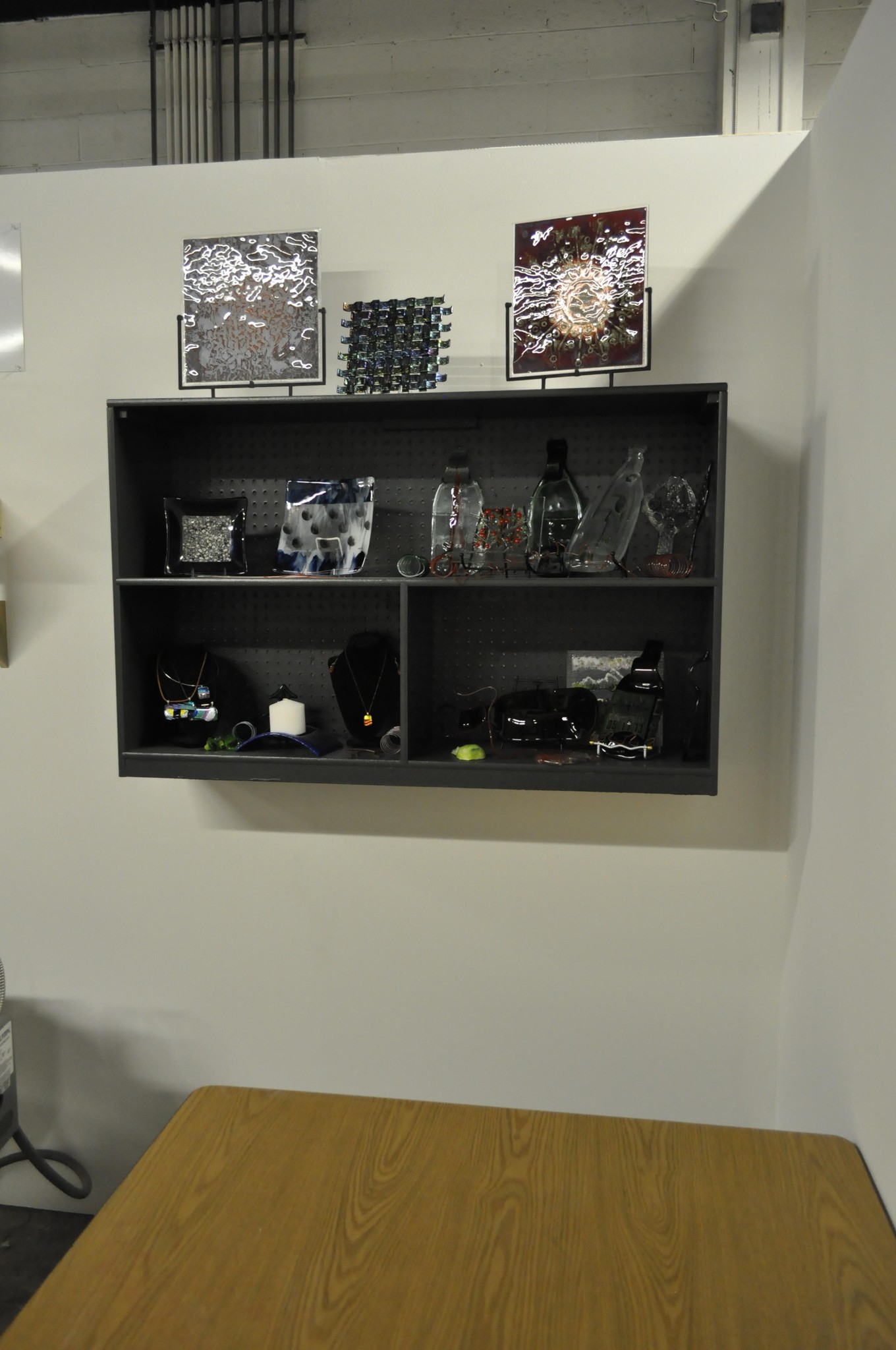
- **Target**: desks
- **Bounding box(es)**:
[0,1085,896,1350]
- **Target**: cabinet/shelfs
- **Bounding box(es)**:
[105,381,727,796]
